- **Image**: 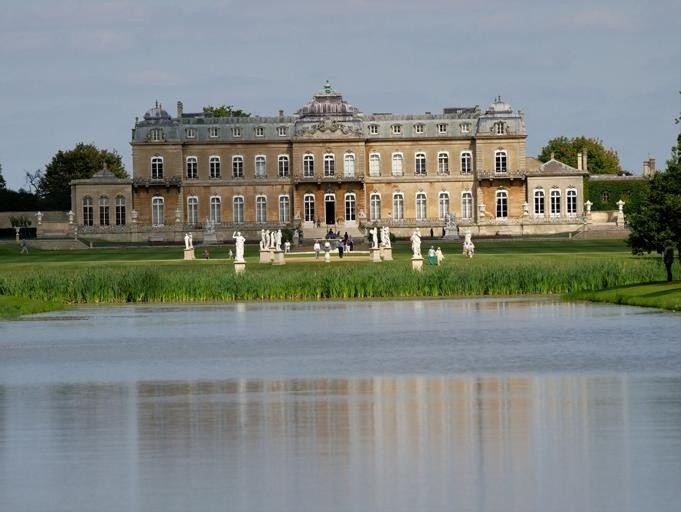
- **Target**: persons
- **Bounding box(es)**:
[385,226,392,248]
[372,227,380,248]
[18,235,30,253]
[260,228,283,251]
[428,245,436,265]
[184,233,190,250]
[380,225,385,246]
[410,228,424,258]
[436,247,445,266]
[232,230,247,262]
[463,228,474,246]
[441,227,446,238]
[284,239,291,255]
[430,228,434,239]
[189,231,193,248]
[315,227,354,258]
